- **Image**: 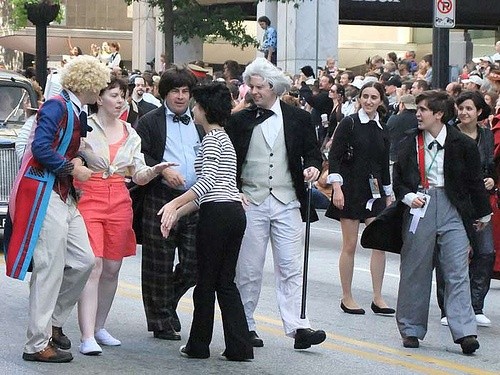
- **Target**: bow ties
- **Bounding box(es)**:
[173,115,191,125]
[428,141,443,150]
[255,110,274,126]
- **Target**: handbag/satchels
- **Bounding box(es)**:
[341,143,354,163]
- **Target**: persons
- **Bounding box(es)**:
[3,54,113,364]
[324,82,395,314]
[0,35,252,115]
[280,41,500,280]
[436,93,500,327]
[221,57,326,349]
[258,16,277,67]
[360,90,493,355]
[75,75,179,353]
[132,65,206,340]
[157,81,254,362]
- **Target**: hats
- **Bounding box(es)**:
[301,64,315,78]
[461,74,483,85]
[348,79,365,89]
[400,94,417,110]
[479,56,492,63]
[186,63,209,79]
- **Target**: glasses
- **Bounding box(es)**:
[331,89,335,93]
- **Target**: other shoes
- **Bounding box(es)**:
[171,312,181,332]
[461,336,479,354]
[440,316,449,326]
[371,301,396,314]
[79,337,102,355]
[294,327,326,350]
[475,314,491,328]
[22,336,74,363]
[250,330,264,347]
[154,327,181,340]
[95,328,121,346]
[51,326,71,350]
[179,345,189,358]
[219,352,228,360]
[403,336,419,348]
[340,298,365,314]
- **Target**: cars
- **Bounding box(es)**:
[0,68,40,230]
